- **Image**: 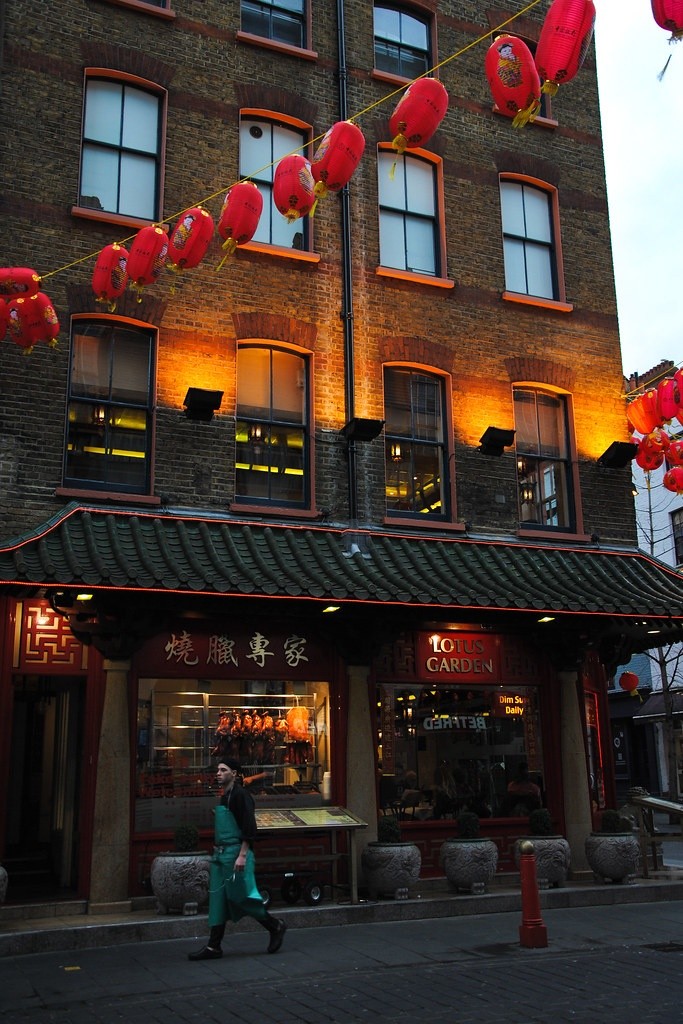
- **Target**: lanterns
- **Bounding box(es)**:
[168,205,214,275]
[218,181,263,254]
[619,671,643,703]
[311,120,365,196]
[125,224,170,302]
[92,242,130,313]
[273,154,316,224]
[627,366,683,496]
[485,34,542,128]
[534,0,596,96]
[652,0,683,85]
[388,77,449,179]
[0,267,61,356]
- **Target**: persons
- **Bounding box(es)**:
[380,769,422,808]
[210,737,274,797]
[506,762,543,809]
[188,758,287,960]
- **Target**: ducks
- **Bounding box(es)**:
[212,710,289,766]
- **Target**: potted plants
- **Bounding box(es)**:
[510,810,574,885]
[582,806,641,880]
[148,822,215,915]
[362,816,422,898]
[441,810,497,885]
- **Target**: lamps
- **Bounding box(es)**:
[54,590,73,606]
[519,477,539,506]
[182,385,224,419]
[479,426,518,458]
[249,426,262,441]
[94,405,105,426]
[389,443,404,462]
[595,440,640,471]
[336,418,385,445]
[517,455,529,477]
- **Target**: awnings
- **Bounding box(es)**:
[632,690,683,725]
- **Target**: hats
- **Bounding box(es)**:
[218,758,243,777]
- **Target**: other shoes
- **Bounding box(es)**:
[188,945,223,961]
[268,920,286,953]
